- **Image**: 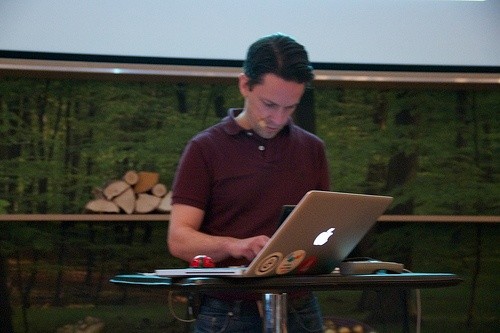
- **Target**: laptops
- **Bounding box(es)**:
[156,190,394,277]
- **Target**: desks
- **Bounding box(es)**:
[109,270,465,333]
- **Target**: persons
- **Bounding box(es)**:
[167,34,330,333]
